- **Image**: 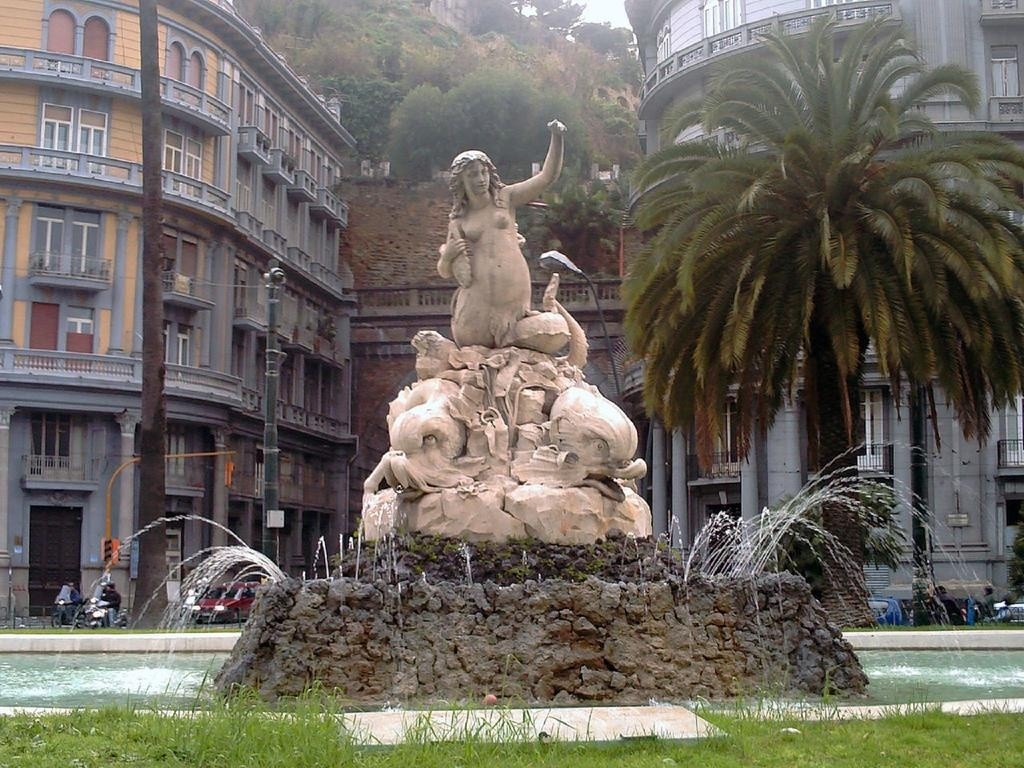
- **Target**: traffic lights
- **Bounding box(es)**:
[110,538,125,565]
[225,463,235,489]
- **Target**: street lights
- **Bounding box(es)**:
[539,250,620,396]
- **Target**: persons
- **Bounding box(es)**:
[982,585,995,617]
[436,117,589,371]
[67,582,81,613]
[101,581,122,613]
[934,585,963,625]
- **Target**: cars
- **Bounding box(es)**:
[192,582,260,623]
[993,601,1024,623]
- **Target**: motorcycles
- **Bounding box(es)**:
[51,597,83,628]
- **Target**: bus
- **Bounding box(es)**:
[865,597,902,626]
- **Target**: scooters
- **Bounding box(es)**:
[75,592,129,629]
